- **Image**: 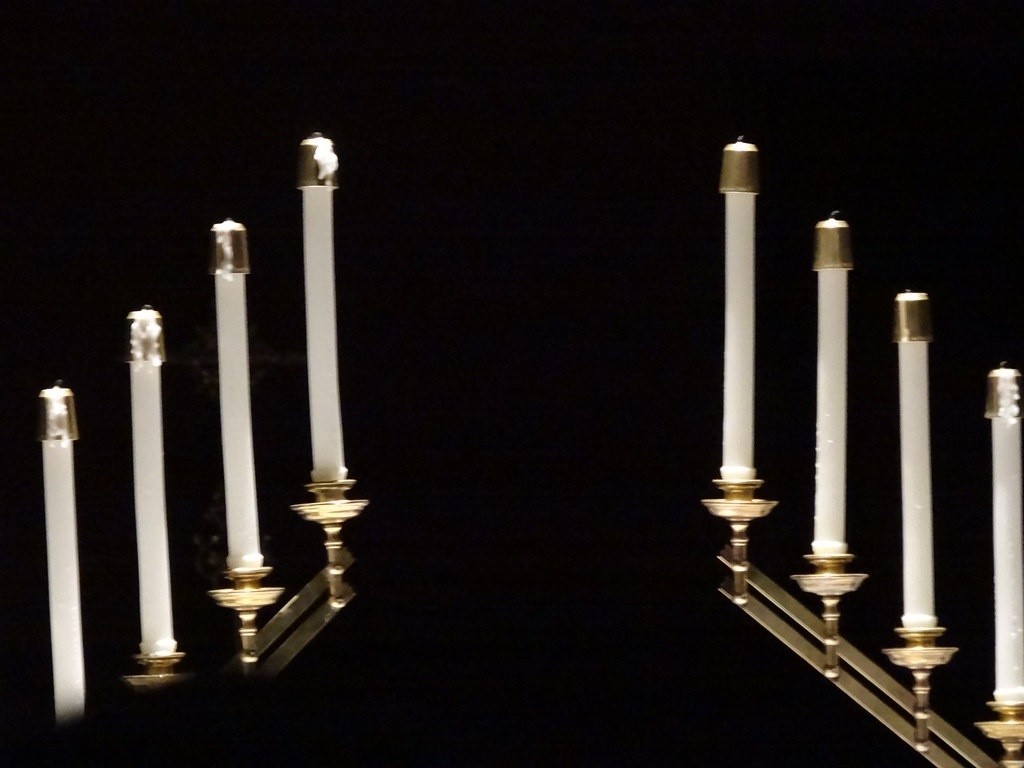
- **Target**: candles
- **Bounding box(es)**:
[295,133,347,484]
[34,378,86,720]
[126,306,179,654]
[213,219,266,568]
[717,137,762,479]
[813,211,854,553]
[891,290,937,629]
[983,362,1024,702]
